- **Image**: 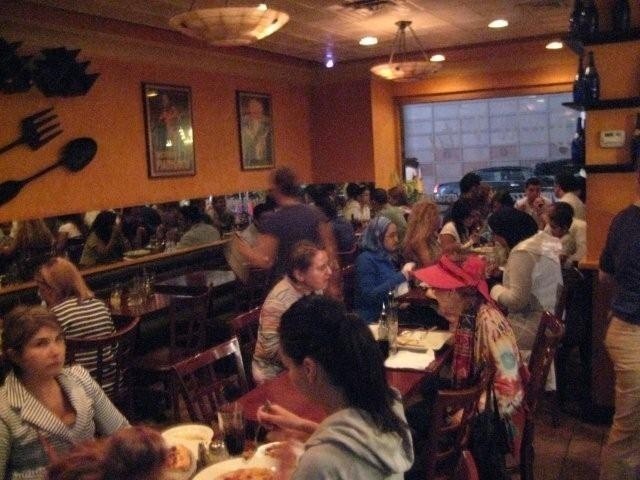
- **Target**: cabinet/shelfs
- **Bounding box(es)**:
[556,24,640,172]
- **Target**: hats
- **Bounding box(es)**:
[409,255,482,289]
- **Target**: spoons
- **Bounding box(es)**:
[1,137,97,207]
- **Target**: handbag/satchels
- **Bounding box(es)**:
[472,407,520,479]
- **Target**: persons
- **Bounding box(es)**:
[252,243,331,384]
[0,195,240,279]
[257,294,414,480]
[0,306,133,480]
[488,206,566,349]
[413,252,530,480]
[595,194,640,480]
[356,217,417,323]
[47,425,168,480]
[38,257,126,401]
[149,91,176,151]
[234,169,588,282]
[242,98,272,167]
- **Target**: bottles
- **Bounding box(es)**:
[254,397,275,445]
[569,1,599,33]
[630,113,640,165]
[614,1,631,32]
[194,435,209,466]
[210,421,229,462]
[386,290,400,351]
[584,50,599,100]
[377,302,390,357]
[572,117,583,163]
[573,51,584,101]
[577,119,586,164]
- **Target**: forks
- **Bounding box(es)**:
[1,99,63,162]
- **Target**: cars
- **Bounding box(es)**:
[431,165,562,201]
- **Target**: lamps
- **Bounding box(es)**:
[370,21,443,84]
[169,0,289,48]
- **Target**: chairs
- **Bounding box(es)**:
[65,244,584,480]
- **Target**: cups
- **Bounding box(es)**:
[109,274,155,310]
[219,403,247,455]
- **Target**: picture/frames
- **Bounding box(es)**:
[236,90,275,171]
[141,82,196,179]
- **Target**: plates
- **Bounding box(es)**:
[119,249,151,261]
[191,458,284,479]
[366,323,381,340]
[161,424,212,449]
[166,451,195,479]
[250,441,304,463]
[382,345,434,371]
[393,329,453,352]
